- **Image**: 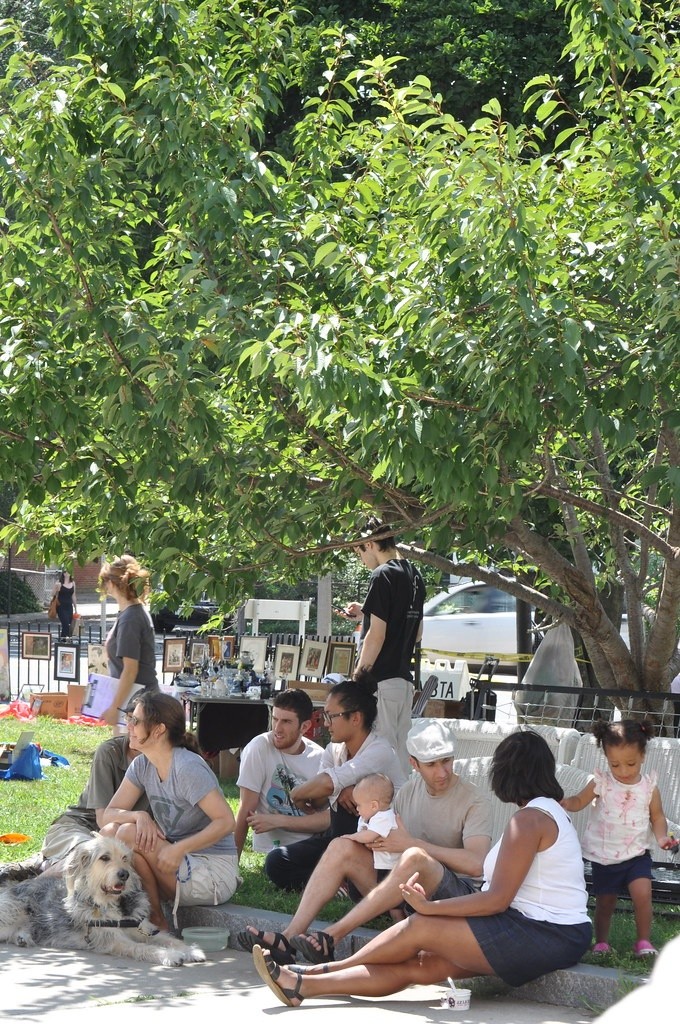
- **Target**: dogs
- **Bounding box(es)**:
[0,834,204,970]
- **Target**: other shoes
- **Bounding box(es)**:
[593,940,611,953]
[633,939,659,957]
[334,879,349,899]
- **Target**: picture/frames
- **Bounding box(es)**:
[238,635,269,674]
[326,641,358,680]
[87,643,111,681]
[274,643,301,682]
[22,632,51,660]
[163,638,187,673]
[54,643,80,682]
[189,639,209,668]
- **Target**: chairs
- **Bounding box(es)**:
[411,675,439,719]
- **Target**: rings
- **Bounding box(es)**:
[379,842,382,847]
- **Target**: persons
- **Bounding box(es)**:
[238,720,495,966]
[252,730,593,1007]
[558,719,677,956]
[53,567,77,642]
[340,774,406,923]
[233,689,328,894]
[98,693,238,936]
[20,688,156,880]
[98,556,159,736]
[332,517,426,775]
[290,670,407,904]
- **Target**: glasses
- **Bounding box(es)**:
[123,714,143,725]
[321,710,358,725]
[355,546,362,557]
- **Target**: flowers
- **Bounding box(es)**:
[170,656,272,700]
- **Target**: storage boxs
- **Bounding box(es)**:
[30,685,88,722]
[288,680,337,702]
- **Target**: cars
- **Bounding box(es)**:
[351,576,656,676]
[150,589,235,632]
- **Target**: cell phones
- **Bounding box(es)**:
[331,604,356,617]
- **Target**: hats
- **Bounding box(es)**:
[406,717,456,763]
[116,688,158,713]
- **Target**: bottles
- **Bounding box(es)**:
[193,660,227,680]
[658,866,667,881]
[272,839,281,849]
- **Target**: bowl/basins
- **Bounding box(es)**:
[181,927,230,950]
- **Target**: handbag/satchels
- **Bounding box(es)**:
[47,601,57,619]
[68,613,86,637]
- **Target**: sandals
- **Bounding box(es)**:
[237,926,297,965]
[283,963,331,975]
[252,944,304,1008]
[289,931,336,964]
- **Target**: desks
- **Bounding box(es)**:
[184,693,269,759]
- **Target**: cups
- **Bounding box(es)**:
[201,681,212,699]
[445,989,471,1010]
[249,686,261,701]
